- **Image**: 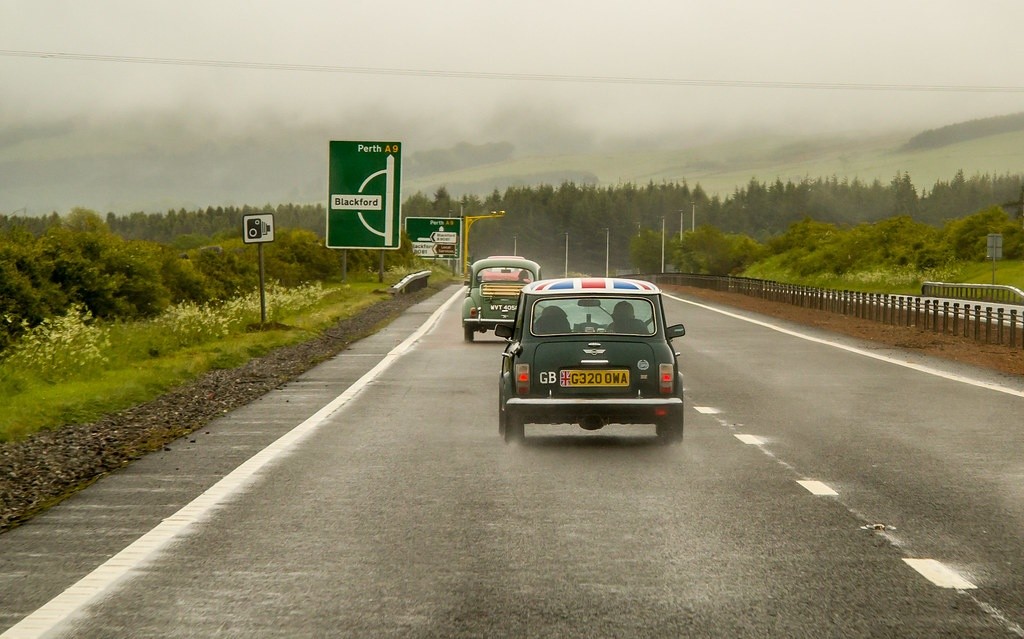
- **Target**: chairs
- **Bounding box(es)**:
[534,312,572,332]
[607,319,650,335]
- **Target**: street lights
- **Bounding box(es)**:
[680,209,683,240]
[691,201,695,231]
[514,236,516,256]
[448,210,452,265]
[459,201,464,273]
[565,232,568,278]
[661,216,665,274]
[606,228,609,278]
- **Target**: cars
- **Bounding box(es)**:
[461,259,542,342]
[494,277,686,444]
[482,256,532,283]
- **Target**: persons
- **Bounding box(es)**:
[607,300,635,334]
[518,270,528,281]
[543,306,567,317]
